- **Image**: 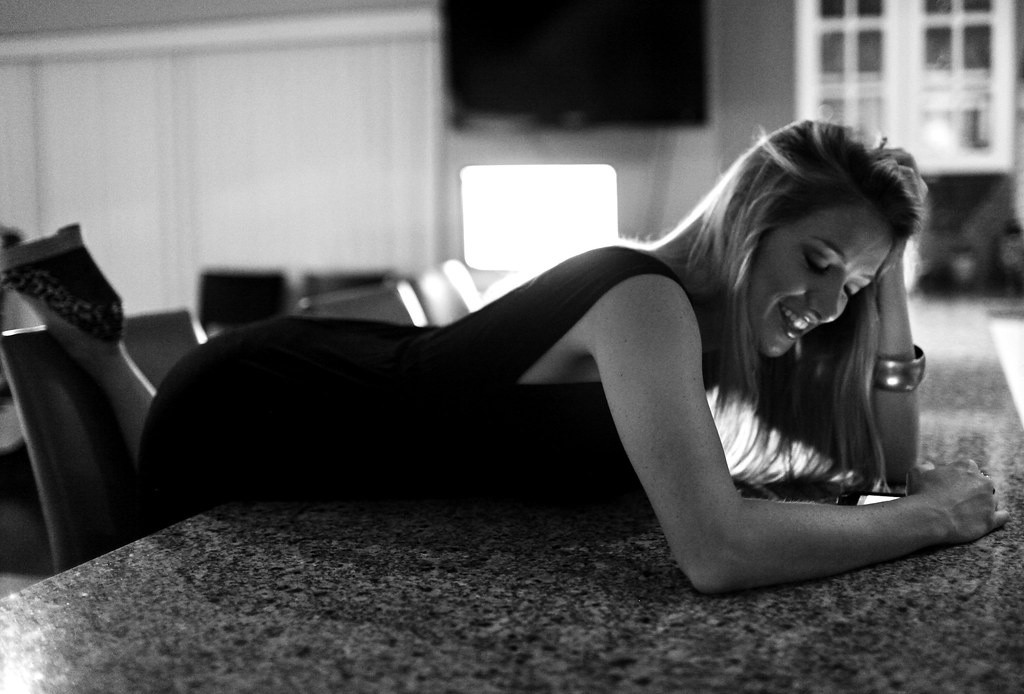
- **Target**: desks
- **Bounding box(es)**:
[2,301,1024,694]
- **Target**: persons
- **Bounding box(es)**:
[1,118,1013,598]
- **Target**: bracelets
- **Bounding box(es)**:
[869,343,927,392]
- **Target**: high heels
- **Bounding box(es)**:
[0,221,125,342]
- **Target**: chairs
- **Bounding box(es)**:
[1,218,479,572]
